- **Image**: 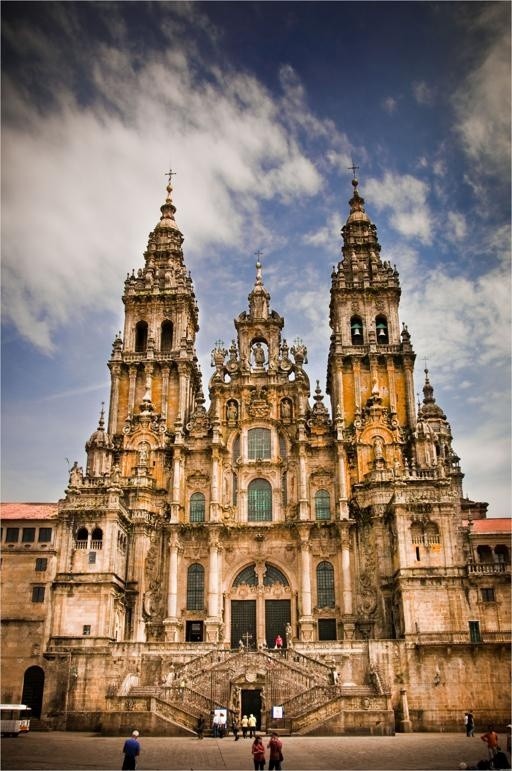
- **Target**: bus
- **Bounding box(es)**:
[0,704,32,738]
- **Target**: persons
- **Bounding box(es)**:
[250,340,266,367]
[68,461,81,489]
[458,746,511,771]
[120,730,141,770]
[278,397,291,419]
[252,736,266,770]
[373,436,384,461]
[480,724,499,759]
[468,709,475,737]
[285,622,292,638]
[198,713,257,741]
[225,399,237,422]
[275,635,282,649]
[267,732,284,770]
[110,462,123,484]
[464,713,469,725]
